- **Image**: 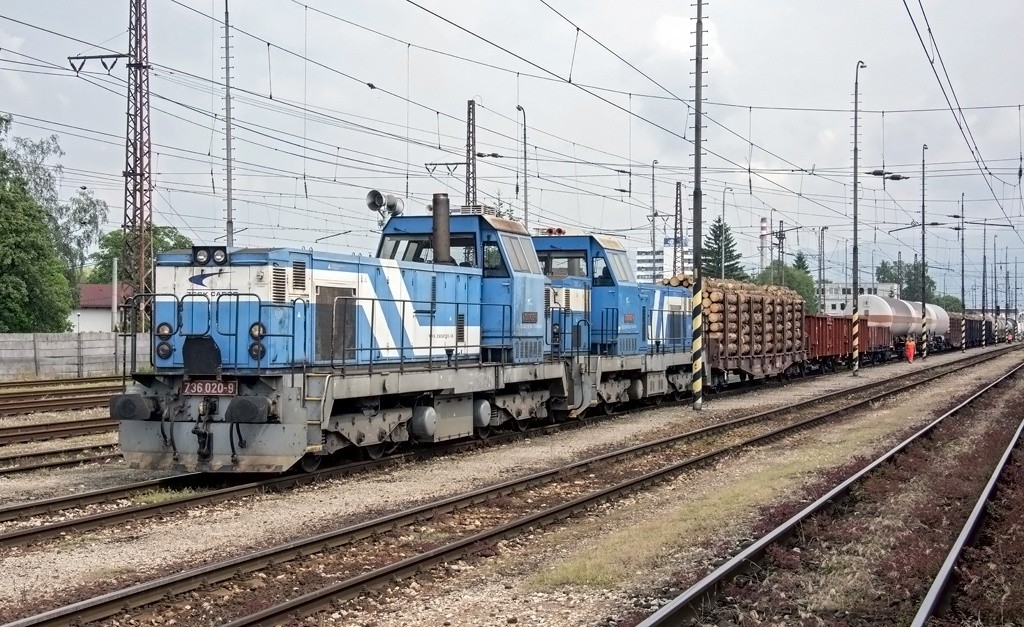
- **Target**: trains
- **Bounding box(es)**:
[109,190,1024,475]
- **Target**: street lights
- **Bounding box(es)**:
[961,191,966,352]
[722,187,734,279]
[852,60,868,373]
[920,144,929,359]
[770,208,778,285]
[982,218,987,350]
[993,235,999,345]
[652,159,659,282]
[516,105,529,229]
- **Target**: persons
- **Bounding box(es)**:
[1008,333,1012,344]
[905,336,917,365]
